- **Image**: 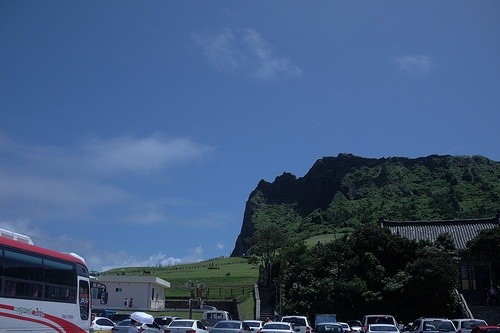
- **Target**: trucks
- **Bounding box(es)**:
[199,311,235,332]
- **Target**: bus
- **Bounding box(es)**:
[0,229,110,333]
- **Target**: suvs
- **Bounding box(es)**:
[281,316,312,333]
[348,320,367,330]
[362,315,398,330]
[314,323,344,333]
[450,319,488,333]
[408,317,458,333]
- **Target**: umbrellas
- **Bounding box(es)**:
[130,312,154,332]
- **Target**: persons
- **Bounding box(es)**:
[399,320,404,333]
[101,296,106,304]
[186,297,206,312]
[266,315,282,322]
[124,298,134,308]
[432,287,456,315]
[81,311,95,320]
[128,319,143,333]
[481,285,500,305]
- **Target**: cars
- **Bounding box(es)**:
[471,326,500,333]
[154,317,185,330]
[365,324,400,333]
[338,322,354,332]
[241,320,266,333]
[111,319,165,332]
[259,322,297,332]
[210,321,243,333]
[89,316,116,332]
[164,319,209,333]
